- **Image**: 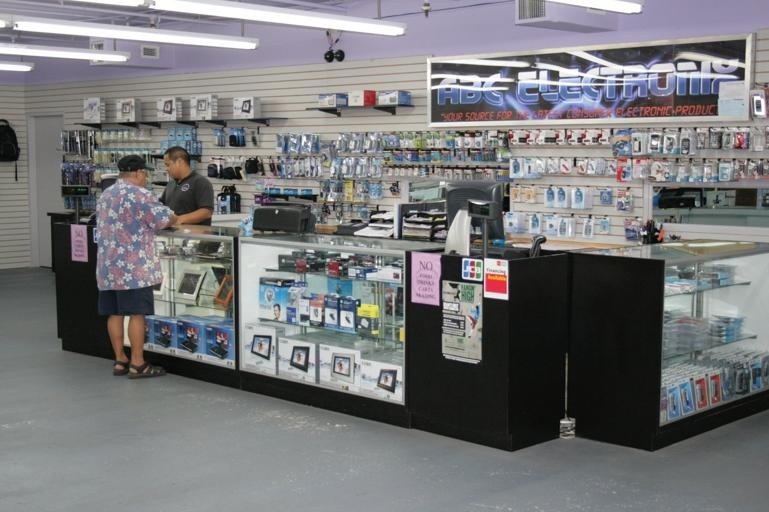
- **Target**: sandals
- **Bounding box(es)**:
[113,361,165,378]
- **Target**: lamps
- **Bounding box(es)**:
[0,0,408,72]
[546,0,643,16]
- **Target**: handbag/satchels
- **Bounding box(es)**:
[217,186,240,214]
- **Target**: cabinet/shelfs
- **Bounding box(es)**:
[567,239,769,450]
[47,208,237,390]
[234,231,568,452]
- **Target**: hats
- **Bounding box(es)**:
[118,155,154,171]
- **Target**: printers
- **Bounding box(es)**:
[252,201,317,233]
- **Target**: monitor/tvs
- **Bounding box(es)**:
[330,352,354,383]
[184,326,199,343]
[241,100,251,113]
[144,320,150,332]
[251,334,272,360]
[159,325,172,337]
[163,100,172,114]
[121,102,131,115]
[175,269,207,300]
[445,180,505,240]
[215,331,229,349]
[290,346,310,373]
[196,99,207,111]
[377,369,397,392]
[214,274,233,306]
[100,173,119,191]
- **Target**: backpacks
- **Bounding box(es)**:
[0,119,21,163]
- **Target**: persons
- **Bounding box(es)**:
[96,154,174,377]
[157,146,213,225]
[272,304,281,320]
